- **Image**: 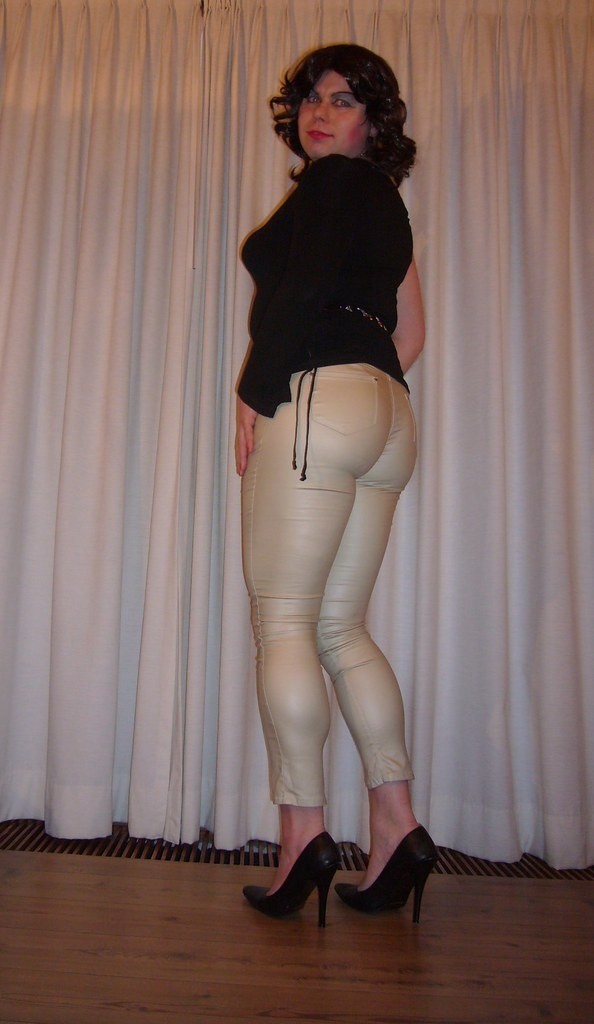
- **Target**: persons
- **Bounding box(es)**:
[236,44,441,929]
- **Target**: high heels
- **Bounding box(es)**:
[242,831,340,929]
[335,824,438,924]
